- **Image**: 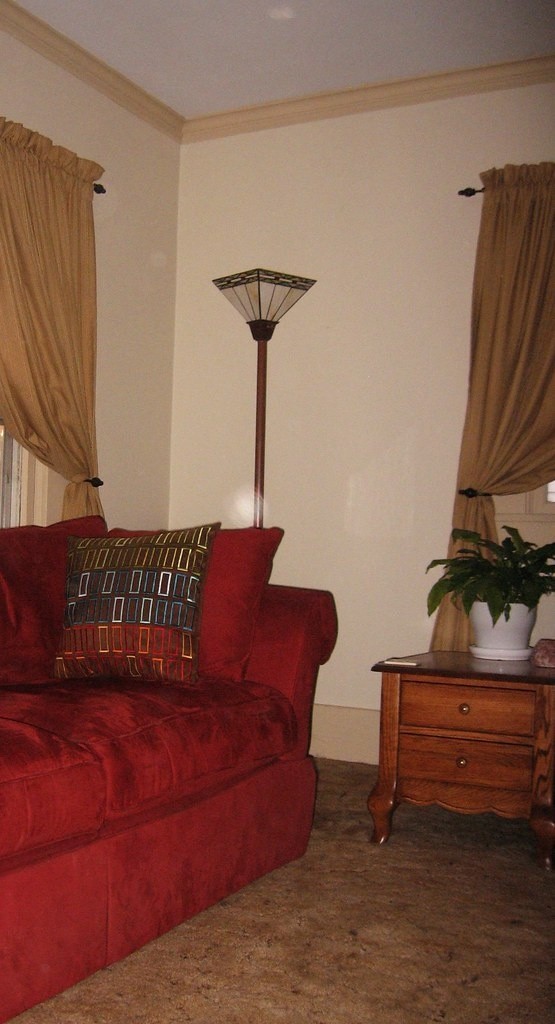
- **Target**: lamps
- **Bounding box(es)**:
[213,268,315,526]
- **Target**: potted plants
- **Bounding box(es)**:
[425,526,555,660]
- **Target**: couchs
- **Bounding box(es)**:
[0,515,339,1024]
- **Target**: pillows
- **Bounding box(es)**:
[53,521,221,685]
[106,526,284,683]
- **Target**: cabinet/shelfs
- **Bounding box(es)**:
[368,651,555,870]
